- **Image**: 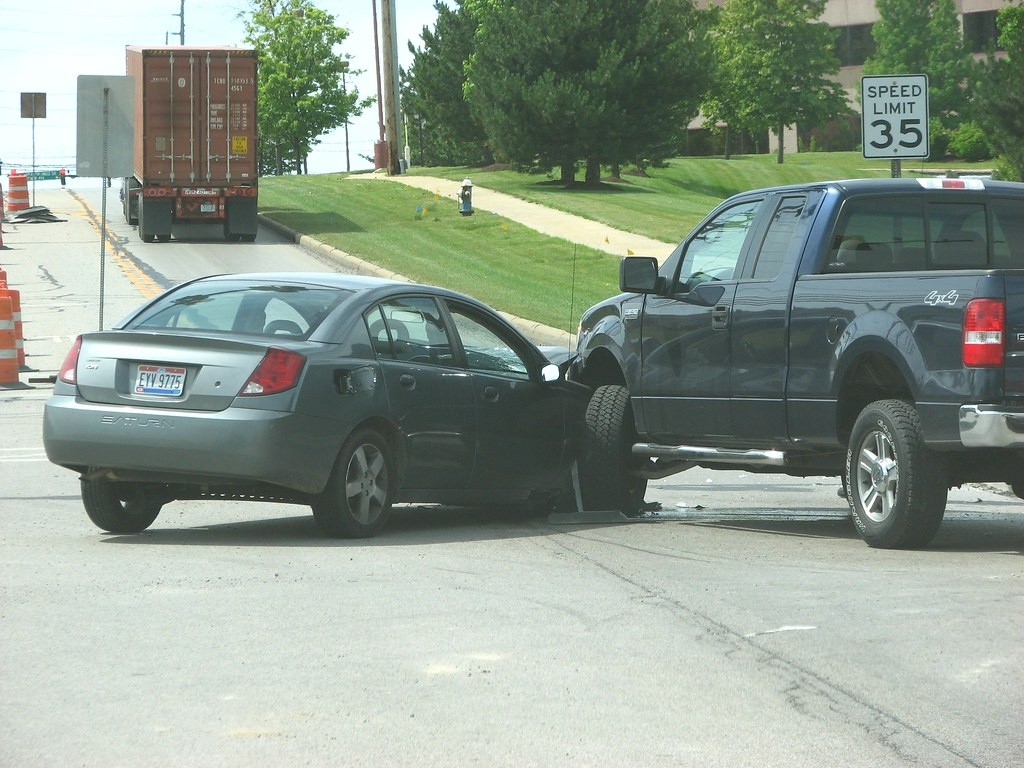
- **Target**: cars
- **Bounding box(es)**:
[42,270,581,540]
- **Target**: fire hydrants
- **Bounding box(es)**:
[456,176,475,218]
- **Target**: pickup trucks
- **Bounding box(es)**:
[567,167,1024,550]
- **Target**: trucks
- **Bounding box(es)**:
[122,44,260,243]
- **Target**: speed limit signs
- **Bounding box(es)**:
[860,73,933,163]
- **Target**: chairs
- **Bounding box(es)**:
[264,320,304,335]
[828,240,892,269]
[935,232,986,267]
[369,319,409,354]
[897,249,932,266]
